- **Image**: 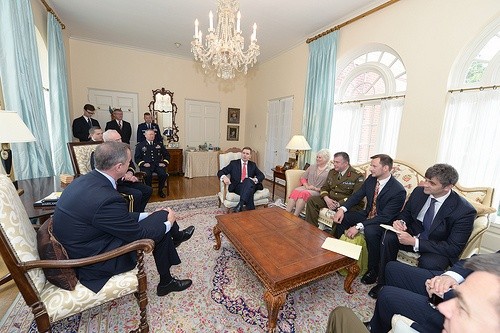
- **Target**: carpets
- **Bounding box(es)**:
[0,192,376,333]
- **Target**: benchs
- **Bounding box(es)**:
[284,160,495,273]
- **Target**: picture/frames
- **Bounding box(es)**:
[228,108,240,124]
[227,125,240,141]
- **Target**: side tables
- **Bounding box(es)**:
[271,167,287,205]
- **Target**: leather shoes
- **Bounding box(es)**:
[363,321,388,333]
[171,226,195,248]
[361,271,377,284]
[157,279,192,296]
[368,284,383,298]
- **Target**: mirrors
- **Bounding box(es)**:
[149,87,180,143]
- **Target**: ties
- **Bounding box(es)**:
[150,141,152,146]
[419,198,437,240]
[118,121,121,128]
[367,182,379,219]
[242,161,246,181]
[148,123,150,129]
[88,118,91,126]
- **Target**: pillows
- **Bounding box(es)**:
[454,191,497,218]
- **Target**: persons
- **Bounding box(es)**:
[137,112,163,144]
[53,141,195,295]
[368,164,477,298]
[89,126,103,142]
[314,250,500,316]
[217,147,265,213]
[287,149,334,216]
[102,129,153,214]
[134,130,169,199]
[72,104,101,141]
[306,151,366,231]
[105,109,132,145]
[329,154,406,284]
[230,129,236,138]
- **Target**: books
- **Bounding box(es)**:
[33,192,62,207]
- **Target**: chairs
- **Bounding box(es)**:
[66,139,147,183]
[215,148,269,214]
[0,175,155,333]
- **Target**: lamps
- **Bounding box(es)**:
[285,135,312,170]
[0,111,38,196]
[190,0,260,81]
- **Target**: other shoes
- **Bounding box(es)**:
[234,204,243,213]
[157,189,166,198]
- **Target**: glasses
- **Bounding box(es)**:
[86,110,95,115]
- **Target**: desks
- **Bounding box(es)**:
[17,176,71,225]
[212,206,361,333]
[184,150,219,179]
[165,148,183,176]
